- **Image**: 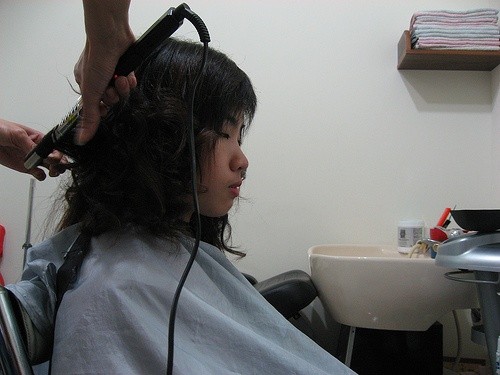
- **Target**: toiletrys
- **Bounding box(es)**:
[397,220,424,254]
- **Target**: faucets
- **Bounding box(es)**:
[418,238,443,248]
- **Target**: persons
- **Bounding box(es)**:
[45,32,365,375]
[0,0,139,182]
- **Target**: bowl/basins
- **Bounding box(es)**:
[450,209,500,232]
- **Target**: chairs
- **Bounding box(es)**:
[0,273,54,375]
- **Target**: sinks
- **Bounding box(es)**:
[307,243,471,332]
